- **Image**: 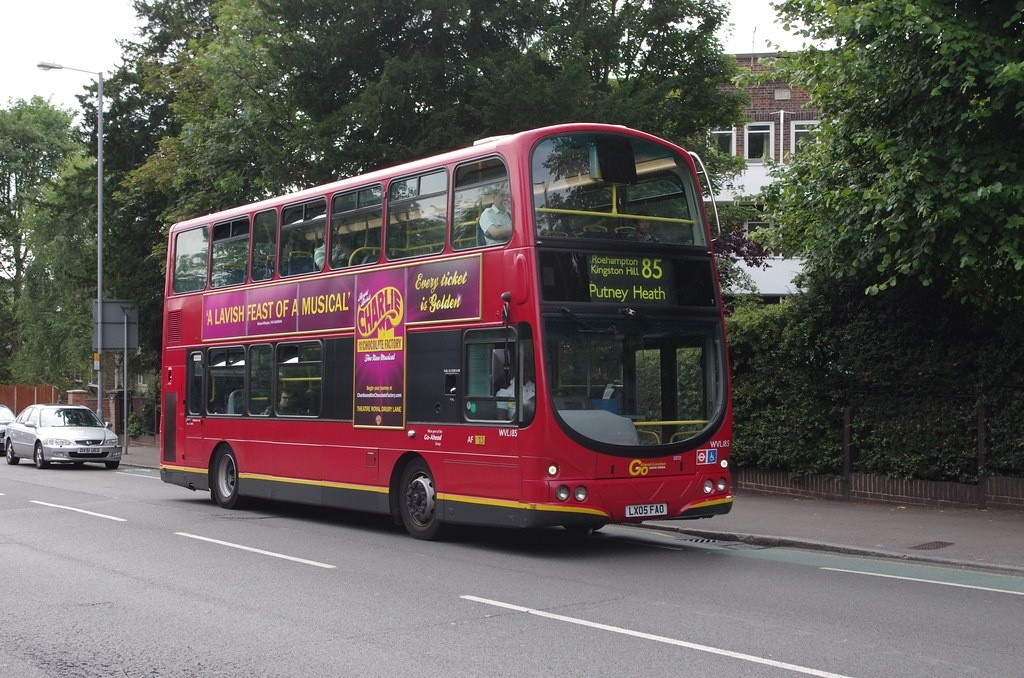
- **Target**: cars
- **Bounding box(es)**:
[0,404,16,456]
[4,403,122,471]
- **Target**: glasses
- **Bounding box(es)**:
[281,397,292,400]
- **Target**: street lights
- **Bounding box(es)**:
[36,62,104,426]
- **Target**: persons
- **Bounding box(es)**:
[495,363,538,422]
[314,227,360,271]
[625,212,660,242]
[478,187,513,246]
[264,391,300,417]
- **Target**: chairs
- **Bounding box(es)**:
[193,227,480,291]
[227,390,266,414]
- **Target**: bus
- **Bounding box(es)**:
[157,123,733,541]
